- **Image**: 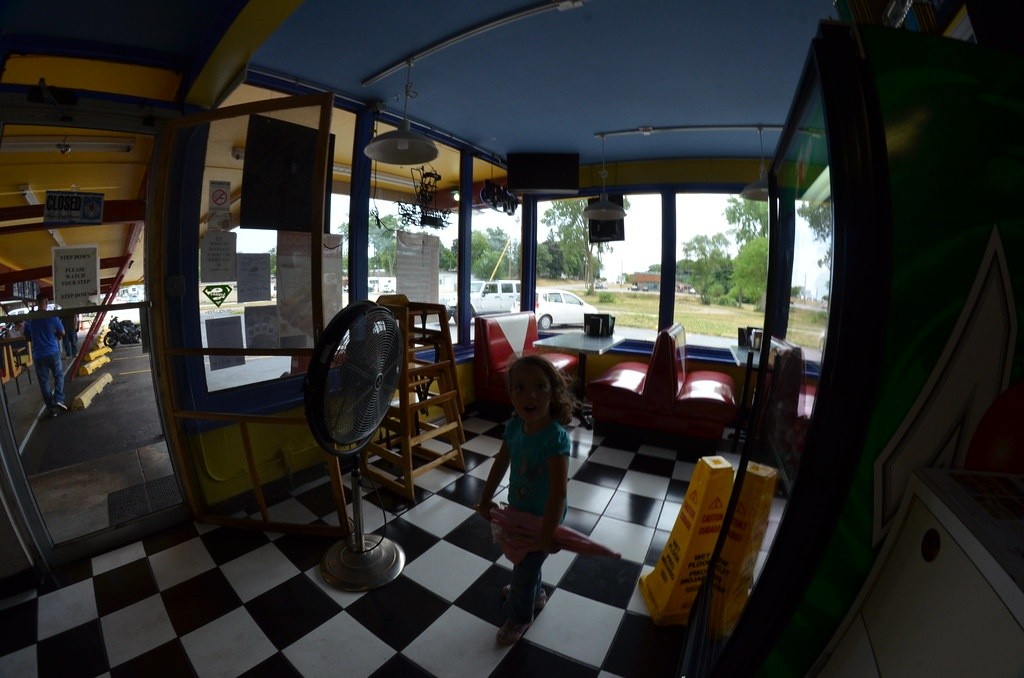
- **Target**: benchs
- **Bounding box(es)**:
[768,335,815,458]
[591,323,736,441]
[472,309,578,415]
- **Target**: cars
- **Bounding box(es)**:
[642,286,649,291]
[342,271,458,295]
[511,287,598,331]
[0,285,143,347]
[631,285,638,291]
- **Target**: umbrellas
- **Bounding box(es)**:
[474,503,621,566]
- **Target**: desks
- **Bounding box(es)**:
[532,334,626,430]
[728,345,773,371]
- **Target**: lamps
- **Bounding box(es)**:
[583,136,627,221]
[741,127,775,200]
[364,55,438,164]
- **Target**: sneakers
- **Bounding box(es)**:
[503,584,546,607]
[497,613,534,646]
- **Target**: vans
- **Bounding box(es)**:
[443,279,520,325]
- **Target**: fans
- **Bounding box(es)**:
[300,300,406,594]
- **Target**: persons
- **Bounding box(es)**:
[21,293,68,417]
[61,313,81,358]
[478,355,570,647]
[8,320,24,339]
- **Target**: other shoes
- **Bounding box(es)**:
[66,356,72,359]
[73,352,79,357]
[47,412,57,417]
[55,402,67,410]
